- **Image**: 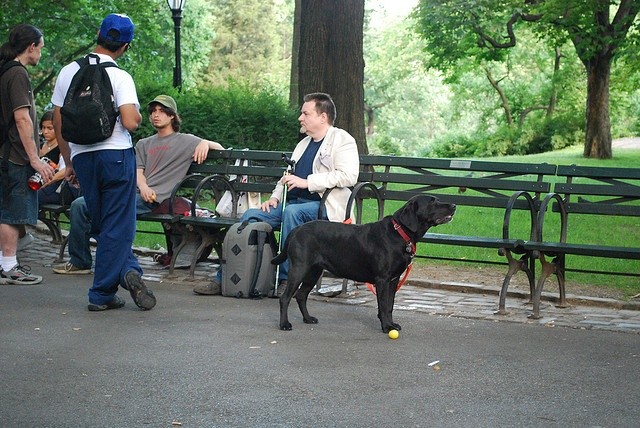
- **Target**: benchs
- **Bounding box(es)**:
[342,155,557,316]
[51,148,231,281]
[38,133,72,244]
[524,163,640,319]
[181,147,373,295]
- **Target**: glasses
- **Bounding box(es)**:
[121,42,131,51]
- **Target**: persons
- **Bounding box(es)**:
[52,13,157,313]
[193,90,361,296]
[52,94,226,275]
[16,109,66,251]
[0,23,54,287]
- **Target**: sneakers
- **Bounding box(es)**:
[52,262,91,274]
[124,270,157,311]
[194,278,222,295]
[88,295,125,311]
[17,232,34,251]
[268,280,299,298]
[0,263,43,285]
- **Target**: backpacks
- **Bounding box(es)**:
[221,217,278,298]
[60,54,126,145]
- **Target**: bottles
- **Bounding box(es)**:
[184,209,211,218]
[27,161,57,191]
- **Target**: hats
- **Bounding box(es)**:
[100,14,134,42]
[148,95,177,114]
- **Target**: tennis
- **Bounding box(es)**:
[389,330,399,339]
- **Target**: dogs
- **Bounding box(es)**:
[270,193,456,334]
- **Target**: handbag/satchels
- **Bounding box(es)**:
[215,148,261,219]
